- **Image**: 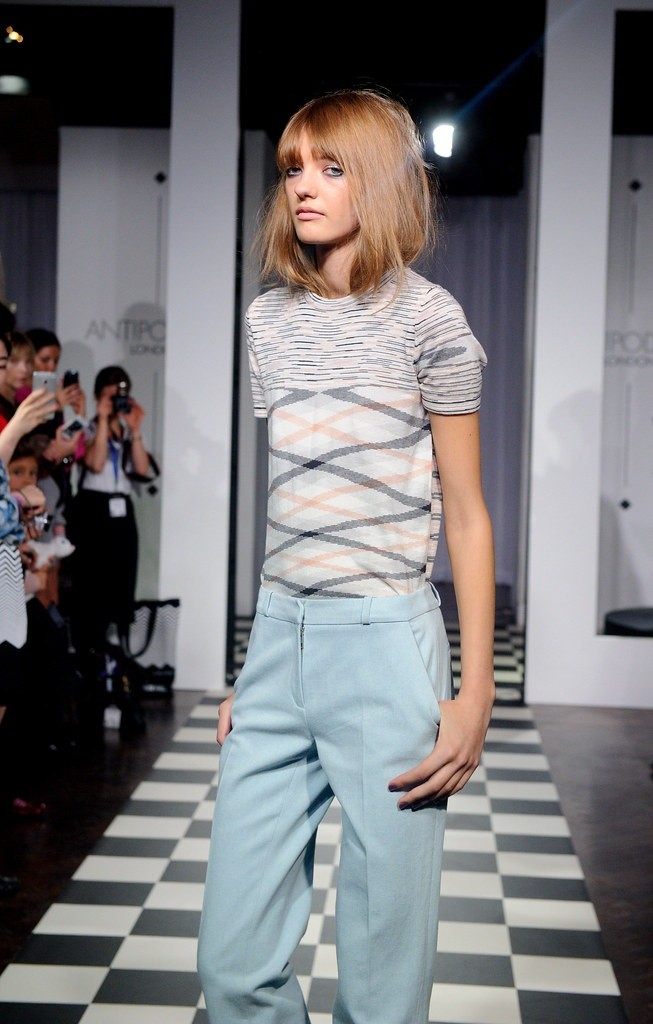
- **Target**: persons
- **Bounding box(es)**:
[197,89,497,1024]
[0,302,152,895]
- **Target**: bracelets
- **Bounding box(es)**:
[129,435,142,442]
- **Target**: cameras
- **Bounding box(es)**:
[110,392,132,414]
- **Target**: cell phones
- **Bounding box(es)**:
[63,370,79,388]
[32,371,57,420]
[60,416,88,440]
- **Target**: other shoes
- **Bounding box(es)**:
[8,800,47,817]
[0,876,22,897]
